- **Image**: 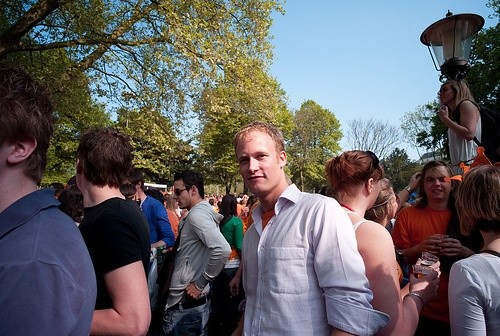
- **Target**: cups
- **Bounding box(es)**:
[244,207,249,213]
[422,252,439,272]
[411,265,433,286]
[434,234,449,254]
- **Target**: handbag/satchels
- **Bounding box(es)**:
[155,251,179,289]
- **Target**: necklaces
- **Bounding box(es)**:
[338,202,355,213]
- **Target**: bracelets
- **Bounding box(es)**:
[194,282,204,292]
[403,293,425,308]
[405,185,412,192]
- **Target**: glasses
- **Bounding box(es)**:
[174,185,199,196]
[438,87,451,95]
[391,195,400,204]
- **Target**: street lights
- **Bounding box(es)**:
[420,9,485,83]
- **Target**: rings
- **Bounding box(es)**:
[436,271,438,276]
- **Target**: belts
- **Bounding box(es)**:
[168,293,212,311]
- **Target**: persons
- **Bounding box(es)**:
[0,68,97,336]
[437,79,482,175]
[48,121,500,336]
